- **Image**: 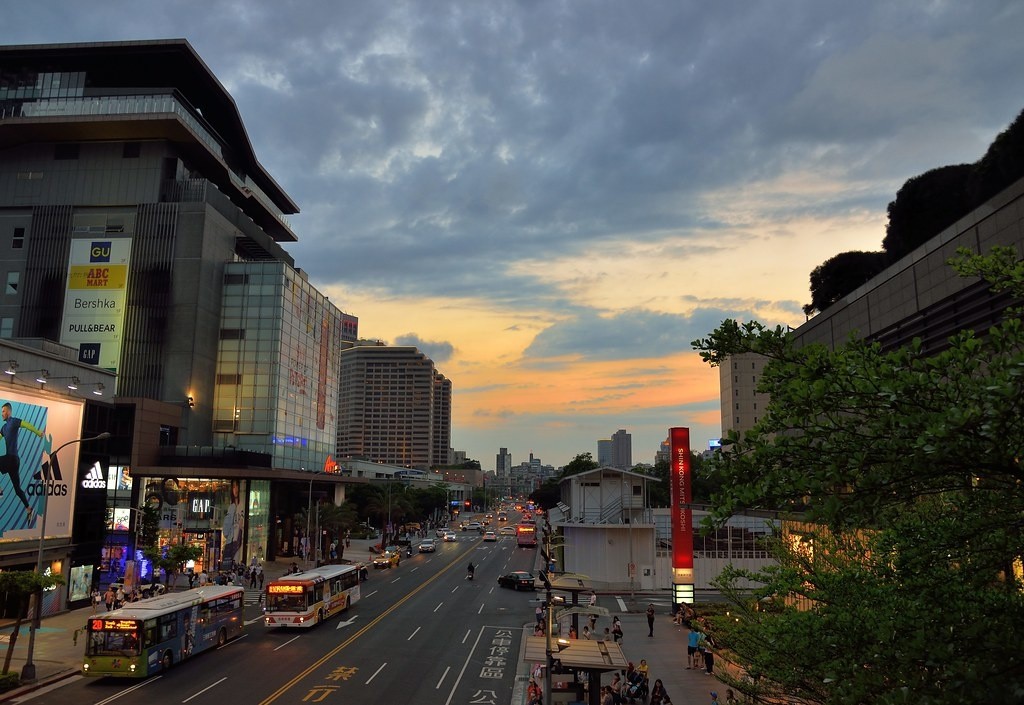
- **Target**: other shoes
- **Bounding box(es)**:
[687,665,715,675]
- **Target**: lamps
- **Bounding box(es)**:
[189,397,195,408]
[16,369,50,384]
[73,382,105,395]
[46,376,80,389]
[0,359,19,375]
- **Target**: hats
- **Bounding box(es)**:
[529,678,535,682]
[710,691,718,697]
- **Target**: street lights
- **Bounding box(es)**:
[304,468,342,568]
[20,431,110,680]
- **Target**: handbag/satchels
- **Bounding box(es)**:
[95,593,102,602]
[617,637,623,645]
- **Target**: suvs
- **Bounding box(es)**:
[461,522,482,531]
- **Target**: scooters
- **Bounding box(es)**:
[468,566,476,579]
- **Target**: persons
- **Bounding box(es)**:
[0,404,45,529]
[346,534,350,548]
[91,587,101,613]
[672,602,695,626]
[568,625,591,640]
[70,572,91,601]
[288,562,303,575]
[587,591,599,634]
[257,545,264,563]
[406,543,413,554]
[221,477,243,569]
[467,562,474,573]
[396,545,403,557]
[645,603,655,637]
[685,625,715,675]
[298,541,336,565]
[103,574,160,612]
[231,560,238,574]
[188,569,237,589]
[534,598,546,637]
[237,555,264,590]
[527,659,673,705]
[710,689,738,705]
[387,518,436,541]
[602,615,623,642]
[119,467,130,490]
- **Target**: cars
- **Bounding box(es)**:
[373,546,402,569]
[444,531,457,542]
[418,538,436,553]
[482,531,497,542]
[497,571,535,591]
[500,526,516,536]
[435,528,451,538]
[461,499,543,525]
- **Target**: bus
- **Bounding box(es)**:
[259,564,362,631]
[73,585,245,679]
[516,523,538,548]
